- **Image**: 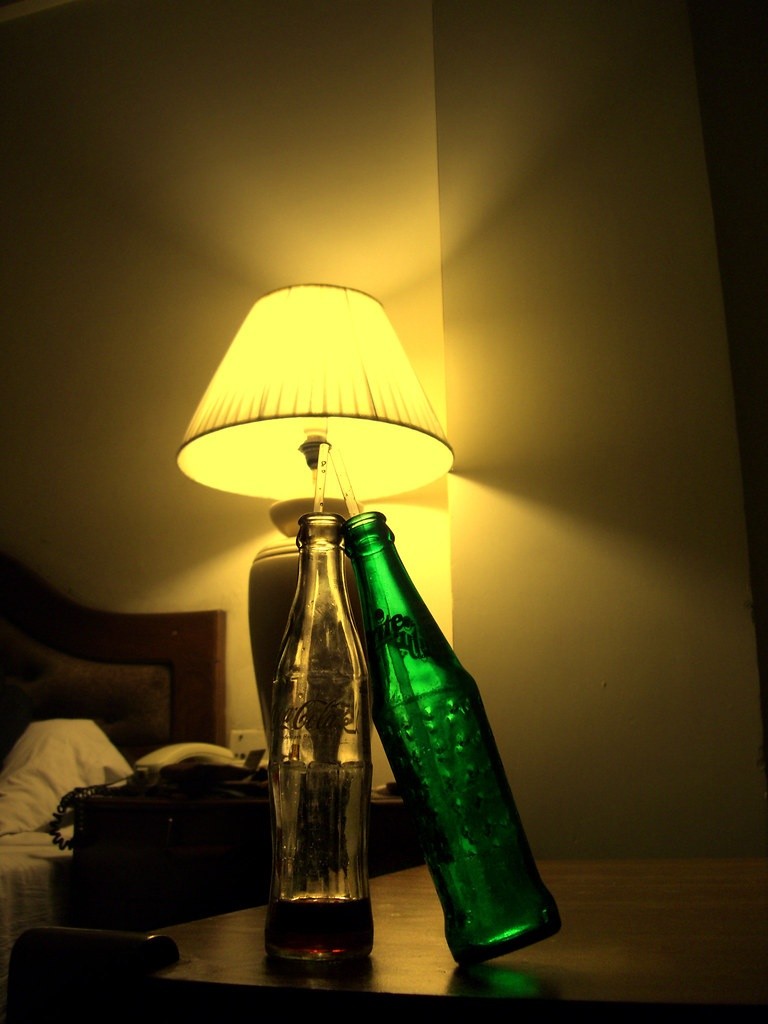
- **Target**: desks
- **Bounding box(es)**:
[120,857,768,1024]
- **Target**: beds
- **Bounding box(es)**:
[0,547,226,1024]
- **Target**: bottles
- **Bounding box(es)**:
[261,510,379,964]
[337,512,566,965]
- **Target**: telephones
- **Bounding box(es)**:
[127,742,245,788]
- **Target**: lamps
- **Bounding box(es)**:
[174,282,456,754]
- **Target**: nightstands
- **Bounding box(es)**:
[71,787,428,934]
